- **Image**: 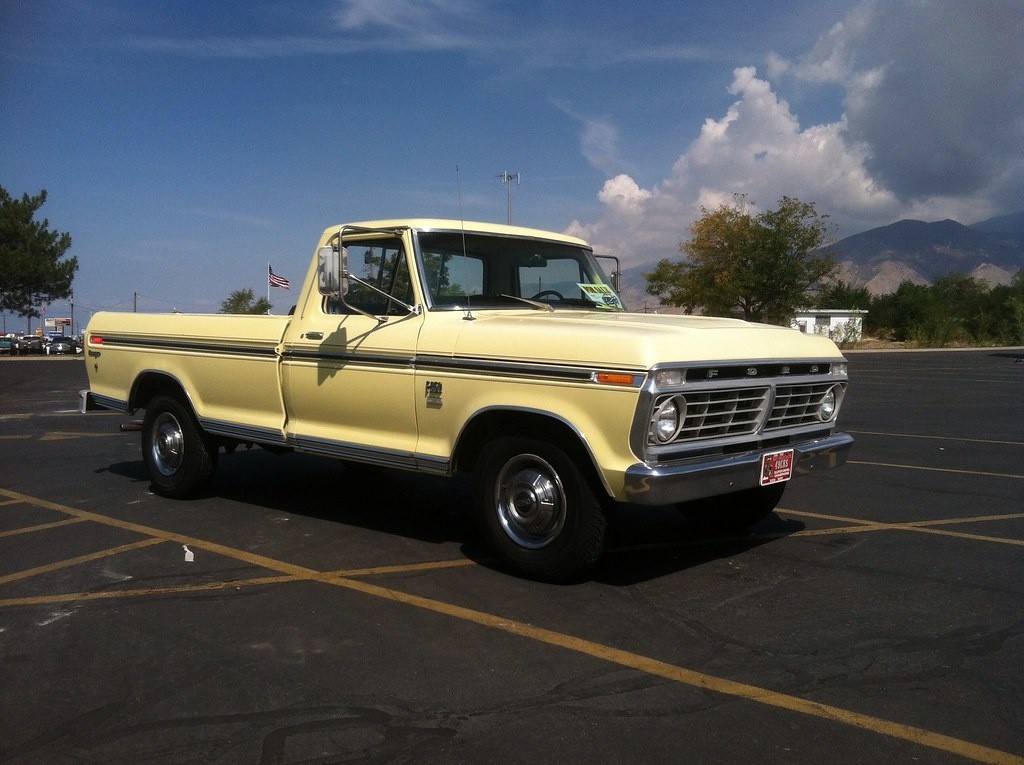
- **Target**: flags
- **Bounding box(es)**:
[269,264,289,289]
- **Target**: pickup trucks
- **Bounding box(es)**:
[78,217,856,584]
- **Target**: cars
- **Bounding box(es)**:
[49,337,76,355]
[0,332,53,356]
